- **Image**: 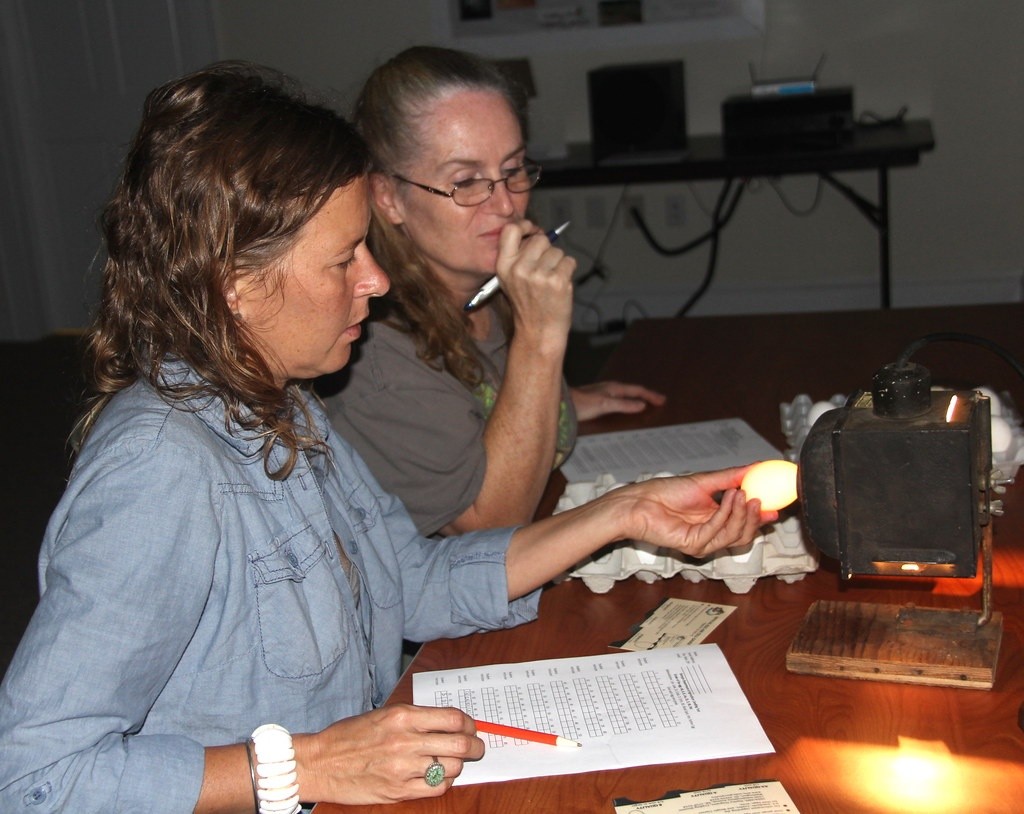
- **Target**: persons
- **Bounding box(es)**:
[1,57,780,814]
[297,43,670,675]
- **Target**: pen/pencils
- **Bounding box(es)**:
[471,719,584,751]
[463,221,572,312]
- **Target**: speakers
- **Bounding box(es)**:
[586,58,688,162]
[722,89,857,159]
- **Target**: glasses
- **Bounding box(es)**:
[392,156,543,208]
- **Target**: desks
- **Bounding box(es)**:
[301,302,1023,812]
[522,114,938,306]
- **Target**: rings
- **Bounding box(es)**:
[424,756,445,786]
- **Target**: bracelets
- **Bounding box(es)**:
[243,738,260,814]
[251,722,302,814]
